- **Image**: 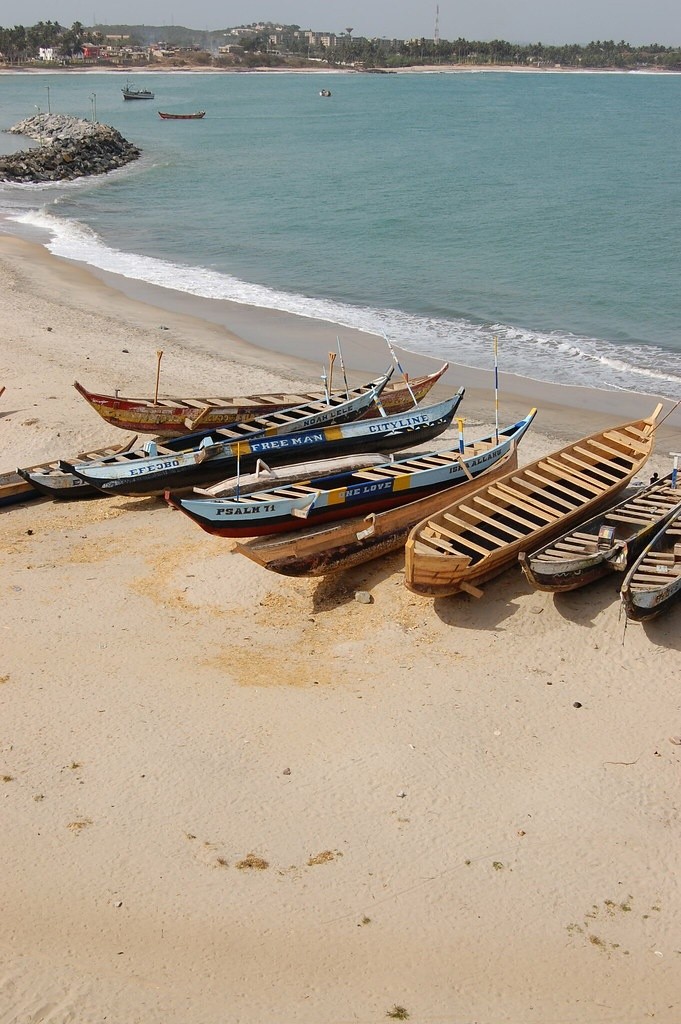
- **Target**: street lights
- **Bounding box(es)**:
[44,85,51,114]
[34,105,42,146]
[88,93,96,125]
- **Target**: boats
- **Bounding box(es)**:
[237,439,516,578]
[620,507,681,622]
[75,363,450,435]
[196,453,393,496]
[319,90,330,97]
[14,362,395,492]
[121,77,155,99]
[0,434,137,501]
[165,407,536,539]
[59,386,466,547]
[158,109,206,119]
[518,457,681,592]
[69,329,464,490]
[404,403,666,599]
